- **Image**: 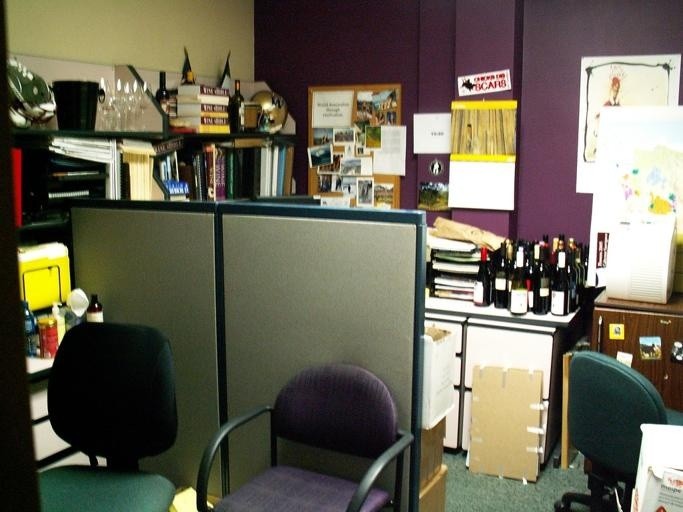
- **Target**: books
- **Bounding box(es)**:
[175,84,231,133]
[427,227,502,300]
[43,135,298,202]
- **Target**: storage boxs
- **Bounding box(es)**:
[422,326,459,429]
[420,416,447,494]
[419,465,448,512]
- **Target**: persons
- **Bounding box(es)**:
[604,77,621,106]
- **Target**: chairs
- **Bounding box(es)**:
[196,361,414,510]
[39,323,179,512]
[555,351,668,511]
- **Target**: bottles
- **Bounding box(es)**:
[576,248,585,285]
[85,294,104,323]
[550,240,571,316]
[583,246,588,282]
[550,238,558,264]
[568,238,576,313]
[506,244,513,266]
[531,239,539,267]
[495,242,508,308]
[24,301,36,357]
[525,246,533,311]
[542,234,553,267]
[37,318,60,358]
[574,242,580,309]
[508,246,528,315]
[230,80,245,134]
[534,242,550,315]
[473,248,492,306]
[155,71,169,118]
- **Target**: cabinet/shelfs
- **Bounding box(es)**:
[116,65,295,198]
[428,298,582,473]
[11,55,115,470]
[591,290,683,414]
